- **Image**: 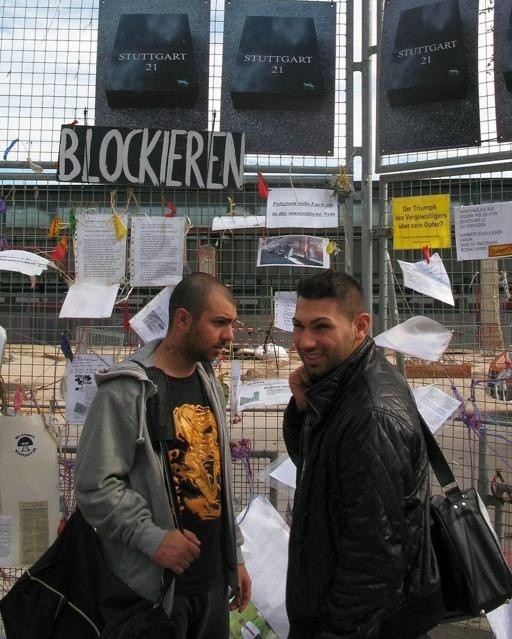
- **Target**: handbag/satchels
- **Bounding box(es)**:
[430,484,512,624]
[0,501,180,639]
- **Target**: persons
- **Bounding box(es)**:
[74,271,252,639]
[282,269,445,639]
